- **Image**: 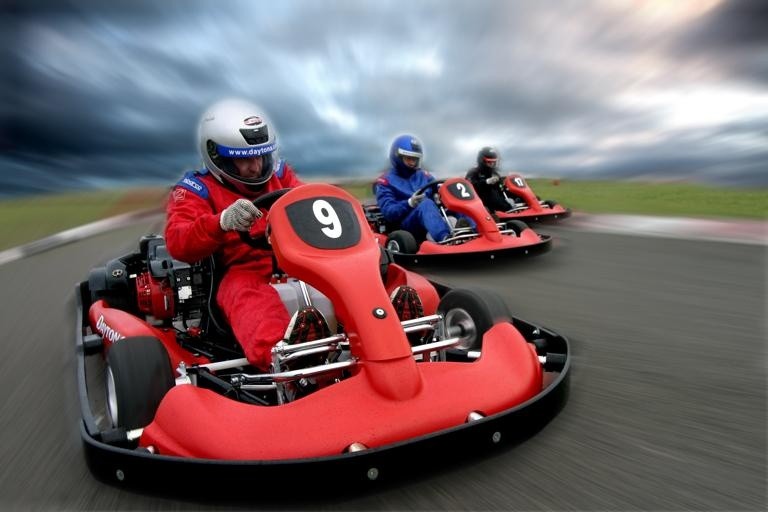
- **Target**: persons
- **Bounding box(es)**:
[167,96,419,406]
[465,146,540,211]
[372,135,478,240]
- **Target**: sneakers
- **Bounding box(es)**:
[455,217,469,229]
[282,305,330,402]
[389,285,422,348]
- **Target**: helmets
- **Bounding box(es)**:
[476,147,498,172]
[196,96,281,189]
[388,135,421,172]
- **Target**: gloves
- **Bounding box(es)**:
[408,188,426,207]
[485,175,500,186]
[220,197,264,231]
